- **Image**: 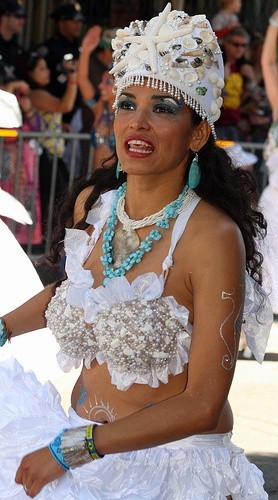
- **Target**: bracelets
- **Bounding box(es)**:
[68,80,76,85]
[0,317,12,347]
[48,424,104,471]
[269,17,278,27]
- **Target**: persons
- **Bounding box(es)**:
[0,0,273,243]
[247,10,278,317]
[0,3,273,500]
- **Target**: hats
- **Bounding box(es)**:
[0,0,27,18]
[109,2,225,140]
[51,2,85,21]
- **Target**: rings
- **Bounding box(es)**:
[24,485,29,492]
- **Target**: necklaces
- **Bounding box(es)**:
[100,182,189,286]
[112,188,196,268]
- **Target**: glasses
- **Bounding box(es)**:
[227,40,249,49]
